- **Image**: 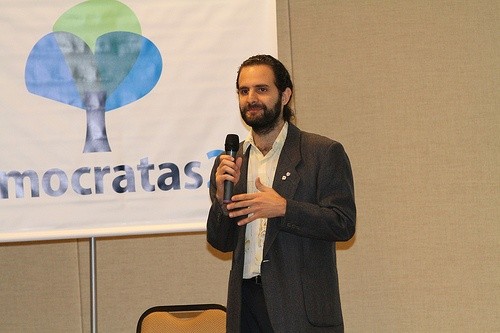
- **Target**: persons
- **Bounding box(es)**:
[207,55,356,333]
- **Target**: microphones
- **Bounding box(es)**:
[223,134,240,204]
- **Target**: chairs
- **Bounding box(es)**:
[136,303,226,333]
